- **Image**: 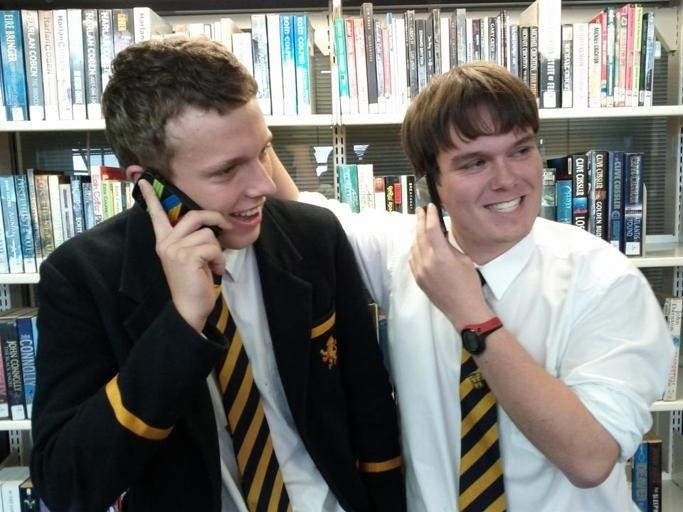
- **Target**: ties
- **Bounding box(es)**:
[204,269,292,511]
[458,270,507,512]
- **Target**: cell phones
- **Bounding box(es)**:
[413,173,447,236]
[131,166,223,239]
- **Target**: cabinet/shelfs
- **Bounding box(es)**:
[0,105,681,509]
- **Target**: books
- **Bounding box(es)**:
[653,290,682,401]
[625,430,662,512]
[337,162,417,214]
[1,465,121,511]
[539,150,644,257]
[0,307,39,418]
[1,166,134,274]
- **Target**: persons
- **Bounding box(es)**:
[27,33,407,512]
[268,60,675,512]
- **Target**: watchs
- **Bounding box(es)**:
[460,316,503,354]
